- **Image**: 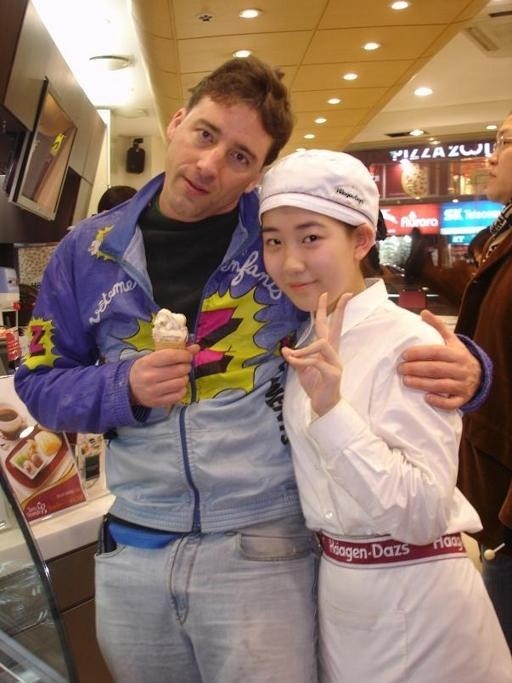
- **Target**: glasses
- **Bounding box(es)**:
[492,135,512,150]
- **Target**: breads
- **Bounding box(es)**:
[34,431,61,453]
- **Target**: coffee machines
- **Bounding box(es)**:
[0,263,21,373]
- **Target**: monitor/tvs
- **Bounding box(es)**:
[8,78,80,223]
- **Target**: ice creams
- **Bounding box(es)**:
[151,308,189,352]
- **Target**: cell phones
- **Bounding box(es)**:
[98,514,116,554]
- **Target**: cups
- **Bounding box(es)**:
[1,402,21,434]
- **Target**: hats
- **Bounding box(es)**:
[259,148,379,232]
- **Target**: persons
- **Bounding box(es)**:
[97,185,139,213]
[260,148,512,682]
[15,55,493,683]
[451,112,511,658]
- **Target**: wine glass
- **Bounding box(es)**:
[425,264,472,314]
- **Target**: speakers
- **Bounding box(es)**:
[127,138,145,174]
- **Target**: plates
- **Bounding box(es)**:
[8,439,56,479]
